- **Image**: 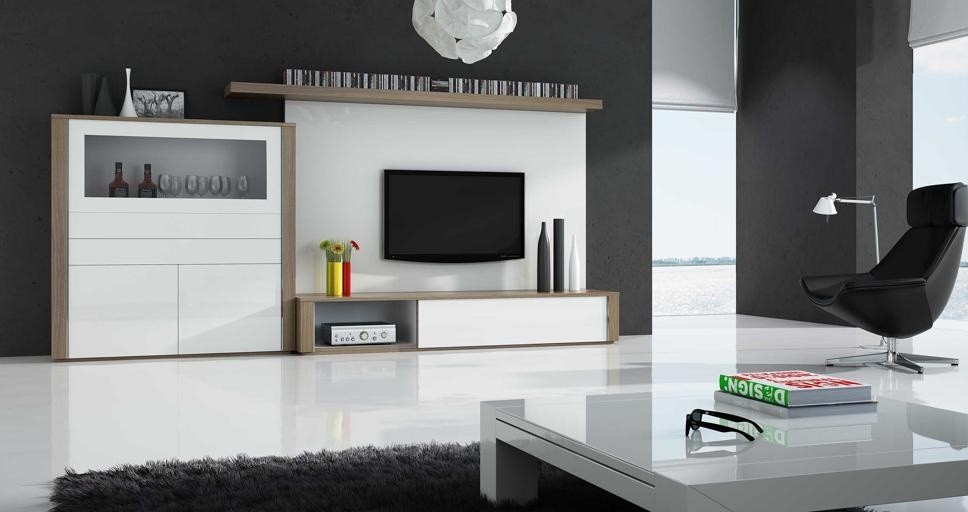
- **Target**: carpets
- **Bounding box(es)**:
[44,438,872,511]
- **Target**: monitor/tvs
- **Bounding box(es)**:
[383,169,525,264]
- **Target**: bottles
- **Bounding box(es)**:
[138,163,157,197]
[109,161,130,198]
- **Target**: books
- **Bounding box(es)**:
[735,422,874,448]
[281,67,578,98]
[721,413,878,429]
[712,390,878,417]
[720,371,877,408]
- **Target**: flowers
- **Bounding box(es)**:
[319,240,360,261]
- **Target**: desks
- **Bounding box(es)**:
[479,375,967,512]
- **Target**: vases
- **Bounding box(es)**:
[551,217,565,292]
[326,262,351,297]
[119,67,139,118]
[537,221,551,293]
[569,235,581,292]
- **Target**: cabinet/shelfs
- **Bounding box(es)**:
[50,113,297,362]
[296,287,621,355]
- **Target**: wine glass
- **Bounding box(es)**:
[158,173,250,199]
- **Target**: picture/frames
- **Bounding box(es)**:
[133,88,185,118]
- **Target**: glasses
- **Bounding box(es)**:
[685,409,764,441]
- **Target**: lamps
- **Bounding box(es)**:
[810,193,890,351]
[409,1,516,64]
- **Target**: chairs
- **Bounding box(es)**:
[801,182,967,379]
